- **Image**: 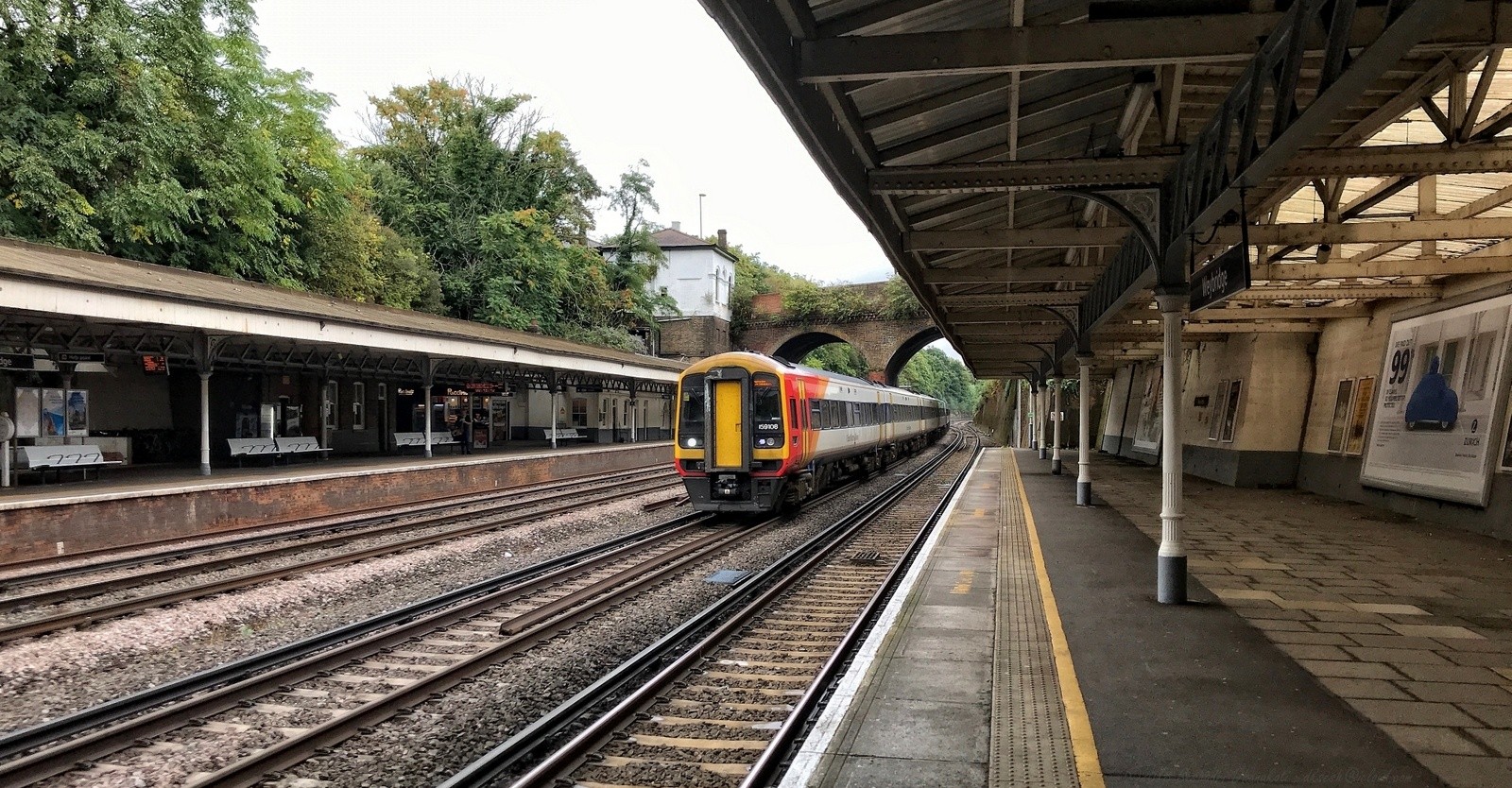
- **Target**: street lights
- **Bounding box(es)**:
[698,191,707,240]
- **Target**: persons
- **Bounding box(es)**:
[454,409,473,455]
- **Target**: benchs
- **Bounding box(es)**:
[226,436,333,469]
[393,431,468,457]
[24,445,122,488]
[544,428,588,446]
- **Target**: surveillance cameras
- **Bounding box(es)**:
[1316,245,1331,264]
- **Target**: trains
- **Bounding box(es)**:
[673,347,950,516]
